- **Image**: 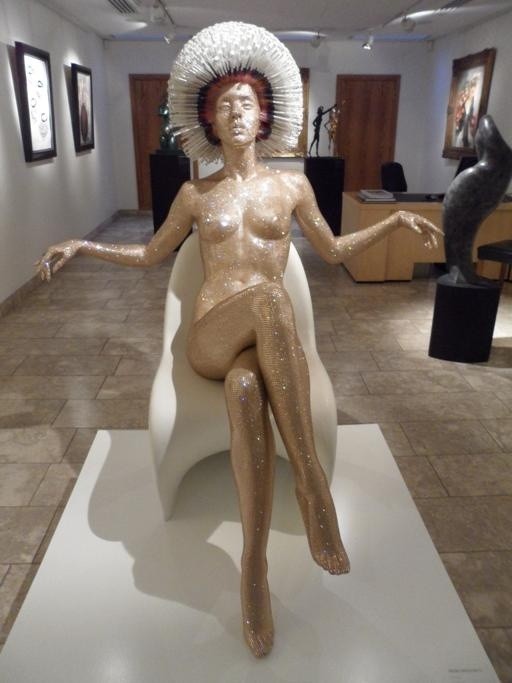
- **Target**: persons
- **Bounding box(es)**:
[36,70,446,660]
[324,108,337,157]
[308,103,336,158]
[453,75,480,149]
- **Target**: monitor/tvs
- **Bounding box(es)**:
[454,157,478,178]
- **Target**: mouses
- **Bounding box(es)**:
[425,193,436,200]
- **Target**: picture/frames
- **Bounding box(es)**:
[440,47,497,160]
[15,40,57,163]
[70,63,95,152]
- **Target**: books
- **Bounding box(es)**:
[361,189,394,198]
[357,192,397,202]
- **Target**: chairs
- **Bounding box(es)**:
[381,161,408,192]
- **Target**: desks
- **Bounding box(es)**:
[339,190,511,284]
